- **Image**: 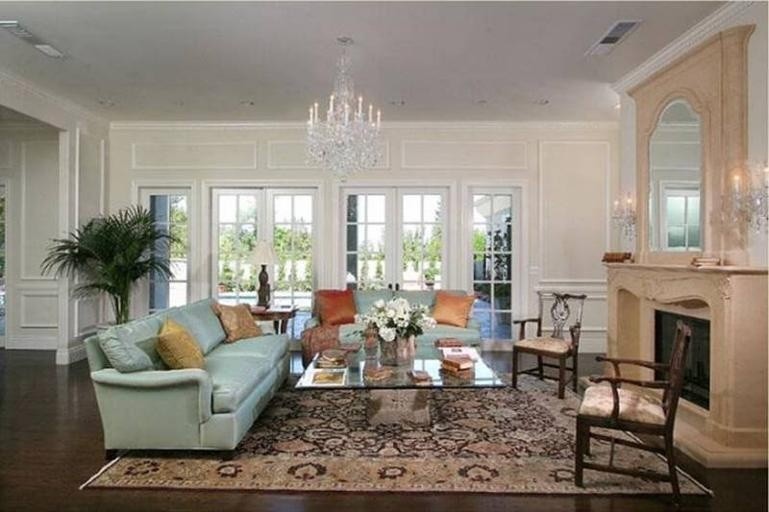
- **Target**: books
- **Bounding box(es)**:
[434,339,475,386]
[690,256,720,267]
[407,370,432,385]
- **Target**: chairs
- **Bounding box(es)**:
[575,320,691,512]
[512,290,587,398]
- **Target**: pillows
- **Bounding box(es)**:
[211,303,264,344]
[314,291,356,324]
[96,316,168,373]
[156,318,206,370]
[431,292,478,330]
[171,301,226,356]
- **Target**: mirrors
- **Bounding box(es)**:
[627,24,756,264]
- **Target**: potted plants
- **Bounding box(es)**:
[38,204,182,335]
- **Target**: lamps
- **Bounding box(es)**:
[723,168,768,248]
[250,237,279,308]
[305,36,385,183]
[610,194,641,240]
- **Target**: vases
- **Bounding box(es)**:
[379,335,416,366]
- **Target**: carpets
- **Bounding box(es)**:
[79,371,715,496]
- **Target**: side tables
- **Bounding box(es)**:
[251,305,299,334]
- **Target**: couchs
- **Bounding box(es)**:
[84,298,291,460]
[301,290,481,371]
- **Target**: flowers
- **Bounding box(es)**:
[352,297,438,343]
